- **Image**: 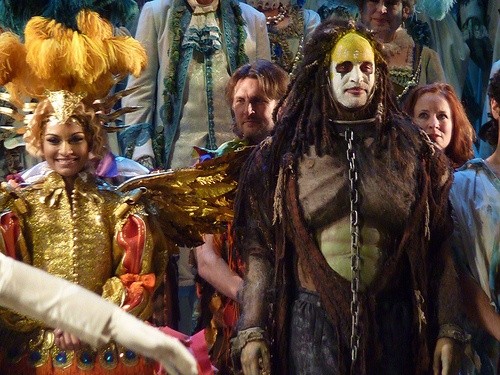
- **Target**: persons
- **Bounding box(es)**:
[0,0,500,171]
[447,68,500,375]
[0,122,238,375]
[229,18,481,375]
[192,60,292,375]
[0,252,200,375]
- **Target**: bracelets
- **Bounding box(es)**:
[230,326,266,355]
[436,323,481,373]
